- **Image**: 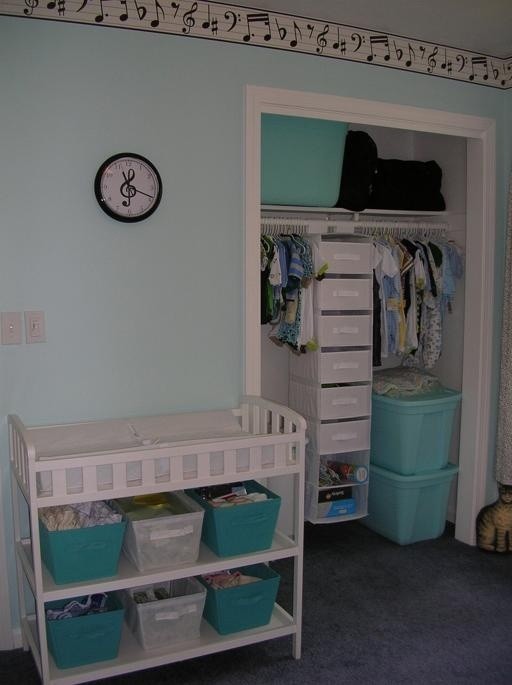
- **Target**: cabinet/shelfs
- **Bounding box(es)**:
[286,234,373,526]
[4,397,319,684]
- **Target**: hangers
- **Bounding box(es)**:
[365,214,453,250]
[253,208,309,252]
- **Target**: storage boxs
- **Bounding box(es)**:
[194,559,285,637]
[260,112,351,208]
[111,497,206,572]
[183,481,283,561]
[365,461,460,547]
[43,590,123,671]
[122,573,210,651]
[368,388,462,476]
[38,506,125,584]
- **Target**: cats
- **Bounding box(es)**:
[475,477,512,555]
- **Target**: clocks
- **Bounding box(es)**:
[91,152,165,223]
